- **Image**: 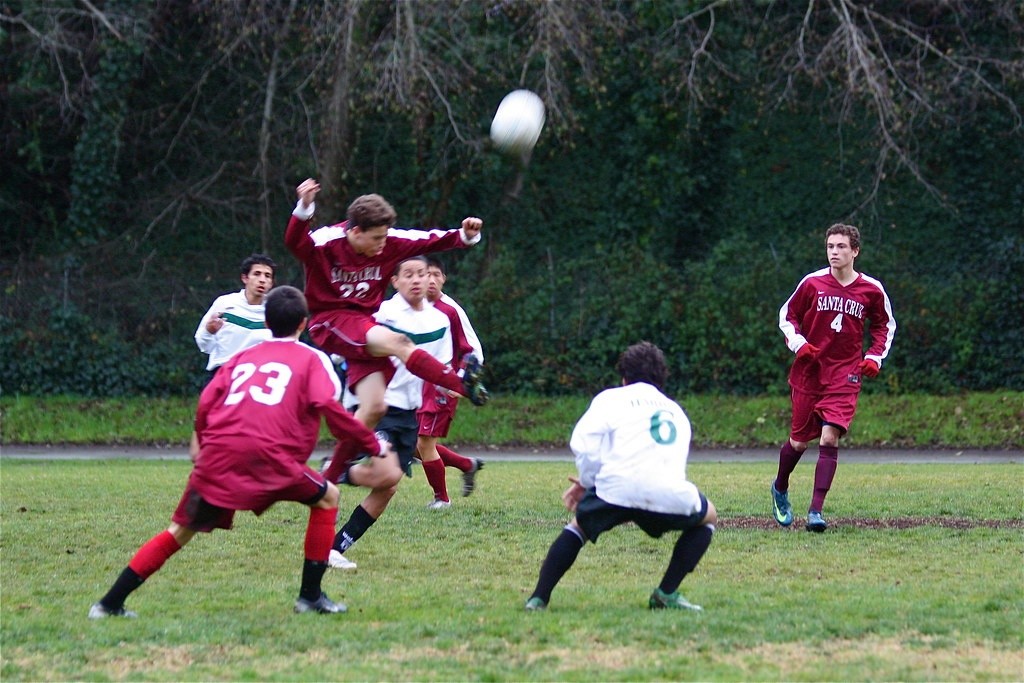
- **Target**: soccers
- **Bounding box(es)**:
[487,89,546,160]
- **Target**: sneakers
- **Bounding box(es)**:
[770,476,794,528]
[462,457,485,497]
[293,592,349,615]
[805,510,828,532]
[462,370,491,407]
[318,457,349,484]
[648,588,702,611]
[427,498,452,510]
[87,601,138,618]
[525,597,544,612]
[327,549,358,569]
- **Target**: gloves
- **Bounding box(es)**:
[794,343,820,368]
[857,359,879,378]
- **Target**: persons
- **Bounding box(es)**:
[525,341,717,613]
[771,223,896,532]
[318,254,479,573]
[284,177,489,486]
[412,257,484,511]
[190,254,278,463]
[88,285,394,620]
